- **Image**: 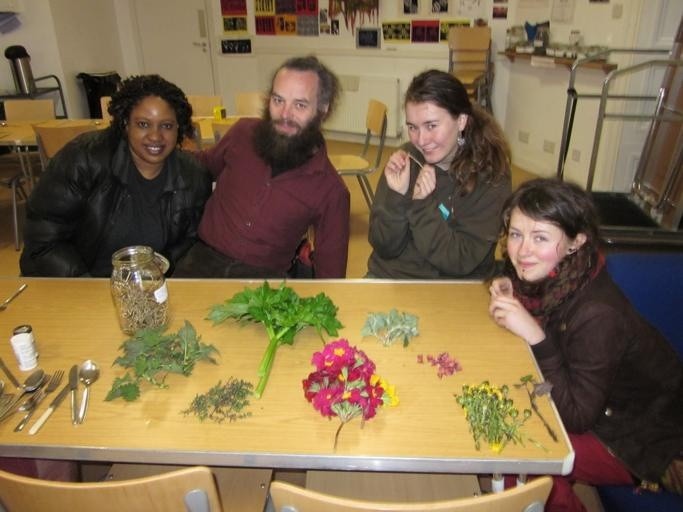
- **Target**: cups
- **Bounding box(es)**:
[213,105,226,121]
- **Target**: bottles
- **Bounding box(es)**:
[10,333,38,372]
[13,324,39,358]
[5,45,36,96]
[505,25,583,53]
[110,246,171,336]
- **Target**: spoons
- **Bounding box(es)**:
[0,283,27,311]
[78,359,99,424]
[0,370,45,418]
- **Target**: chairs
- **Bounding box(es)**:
[269,470,554,512]
[448,28,495,115]
[328,99,389,212]
[1,464,274,512]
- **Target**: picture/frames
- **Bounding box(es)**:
[355,27,382,50]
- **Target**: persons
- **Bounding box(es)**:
[368,70,512,280]
[489,180,682,512]
[19,74,212,278]
[174,58,349,279]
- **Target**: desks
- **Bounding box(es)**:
[1,274,576,495]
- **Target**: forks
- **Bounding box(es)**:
[15,370,64,431]
[0,374,51,421]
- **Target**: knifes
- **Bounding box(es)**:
[28,366,78,435]
[69,365,78,425]
